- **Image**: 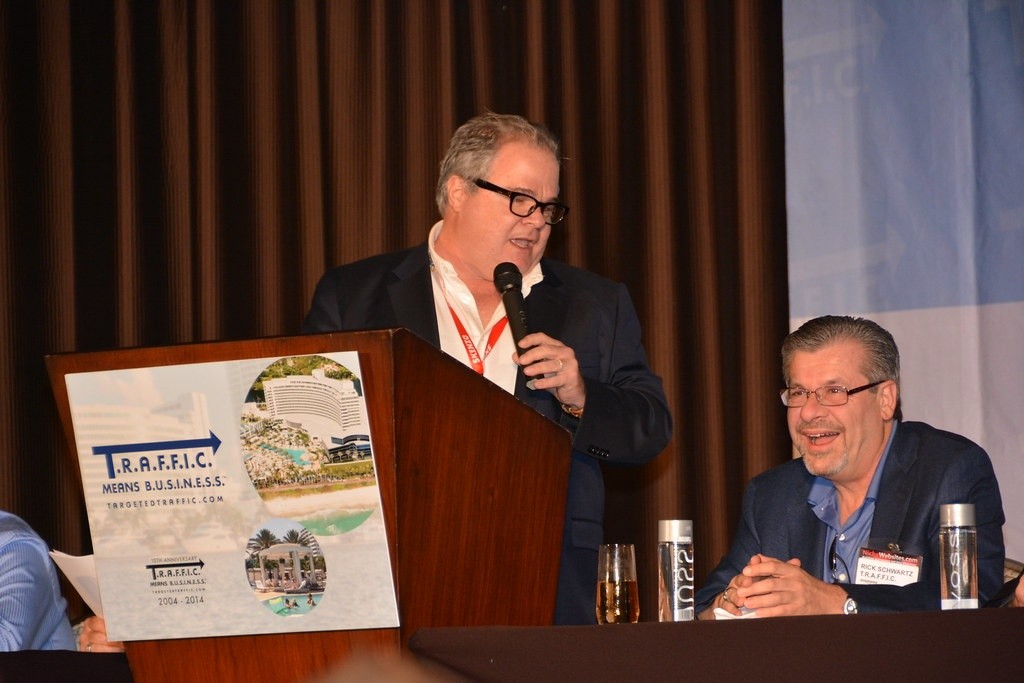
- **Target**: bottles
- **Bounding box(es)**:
[657,519,695,623]
[938,503,979,610]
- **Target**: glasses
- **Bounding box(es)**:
[469,175,570,225]
[779,380,886,407]
[829,537,851,584]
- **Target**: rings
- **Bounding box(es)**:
[86,645,90,652]
[558,358,563,370]
[723,587,733,601]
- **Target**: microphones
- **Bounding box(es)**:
[493,262,545,392]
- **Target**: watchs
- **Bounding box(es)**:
[841,594,857,615]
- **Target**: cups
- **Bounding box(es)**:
[596,543,640,623]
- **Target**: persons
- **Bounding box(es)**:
[268,570,315,608]
[299,112,673,626]
[71,616,127,653]
[982,568,1024,607]
[0,510,77,651]
[695,316,1005,620]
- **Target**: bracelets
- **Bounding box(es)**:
[560,404,583,417]
